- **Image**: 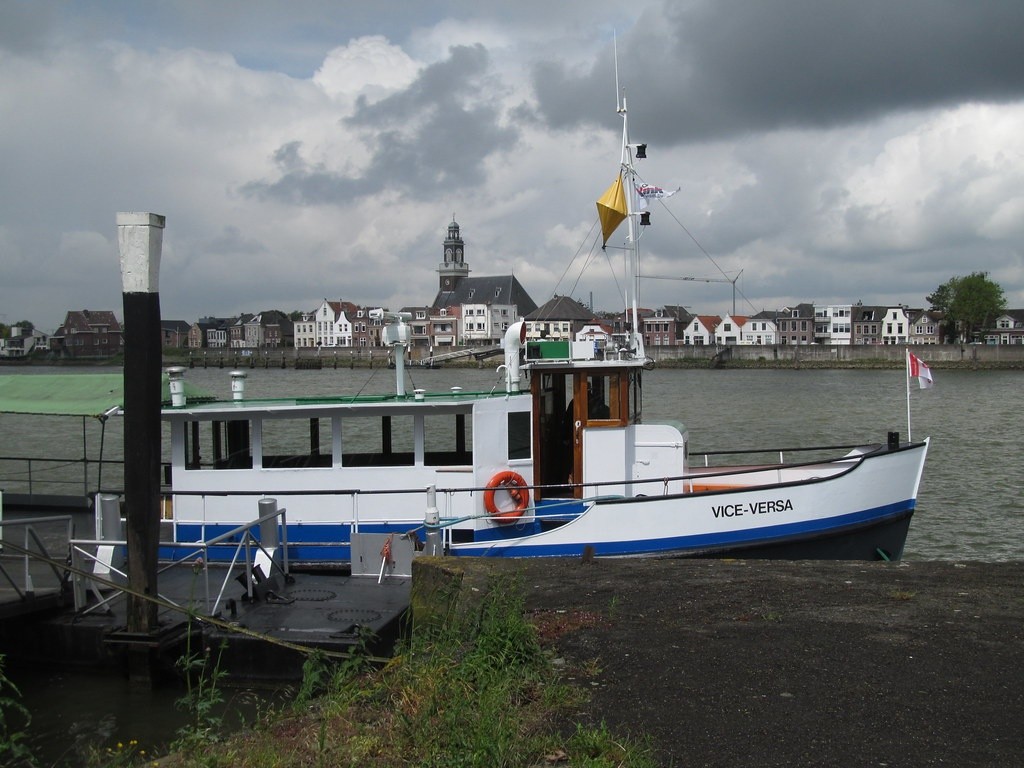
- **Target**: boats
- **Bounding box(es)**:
[1,31,930,579]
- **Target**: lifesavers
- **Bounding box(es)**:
[482,471,529,525]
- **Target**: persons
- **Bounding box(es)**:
[565,382,596,439]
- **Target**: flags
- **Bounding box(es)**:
[908,352,932,389]
[639,183,681,210]
